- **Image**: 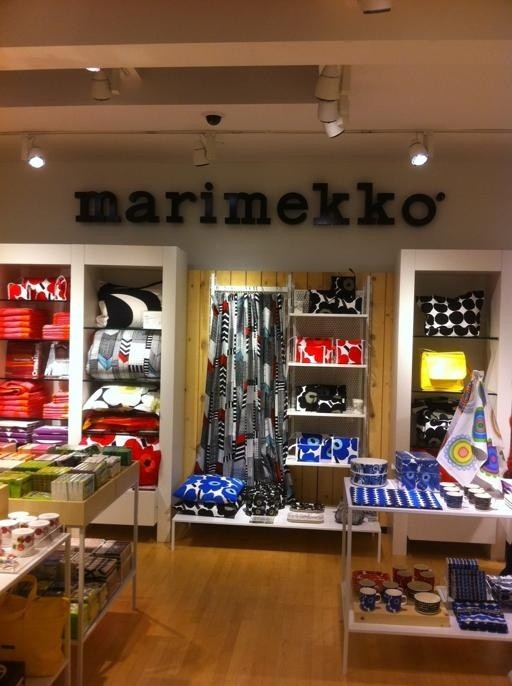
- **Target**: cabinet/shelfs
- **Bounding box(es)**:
[394,249,508,463]
[340,477,512,678]
[286,272,375,470]
[0,526,73,685]
[0,241,184,545]
[0,458,148,685]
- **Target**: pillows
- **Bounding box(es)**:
[170,471,245,503]
[171,494,245,519]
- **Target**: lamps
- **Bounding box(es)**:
[87,67,114,102]
[314,65,346,138]
[360,0,393,15]
[23,128,435,170]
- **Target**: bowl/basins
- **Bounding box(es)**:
[413,593,441,616]
[407,581,432,600]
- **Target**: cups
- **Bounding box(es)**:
[353,399,364,415]
[0,511,61,558]
[359,578,408,613]
[439,482,492,511]
[392,562,434,590]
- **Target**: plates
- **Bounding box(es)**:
[354,571,390,585]
